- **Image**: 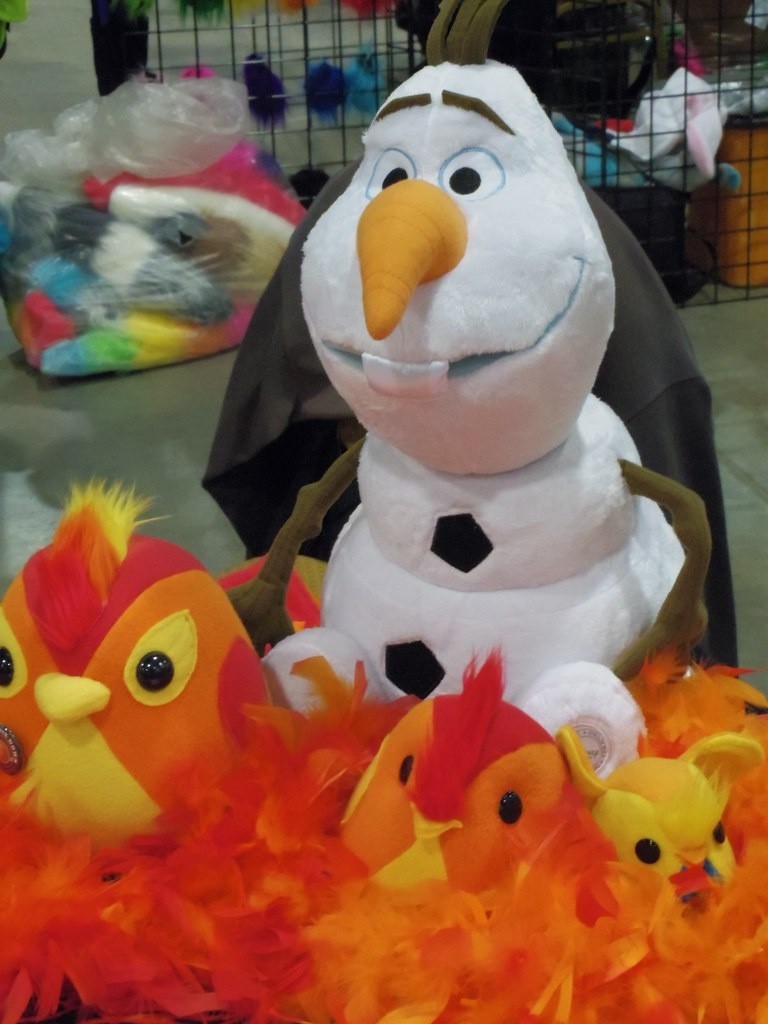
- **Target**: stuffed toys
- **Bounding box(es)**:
[1,0,768,1023]
